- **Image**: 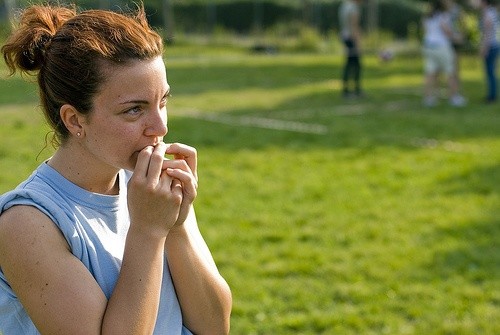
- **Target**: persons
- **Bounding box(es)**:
[339,0,367,99]
[0,5,233,335]
[443,0,466,89]
[422,0,466,106]
[473,0,500,102]
